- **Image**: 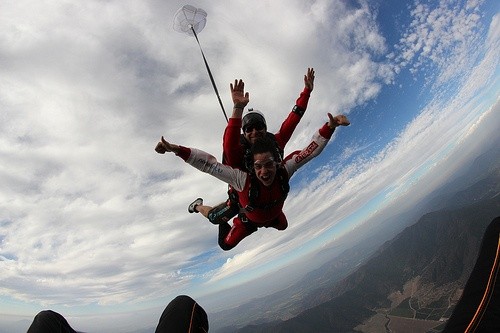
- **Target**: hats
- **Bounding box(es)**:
[241,109,266,127]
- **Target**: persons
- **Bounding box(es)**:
[188,67,316,225]
[155,113,351,252]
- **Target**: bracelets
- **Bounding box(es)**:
[234,104,244,110]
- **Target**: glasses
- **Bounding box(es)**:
[254,158,277,170]
[243,122,267,133]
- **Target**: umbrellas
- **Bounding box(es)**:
[173,5,207,38]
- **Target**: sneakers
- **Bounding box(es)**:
[188,198,203,214]
